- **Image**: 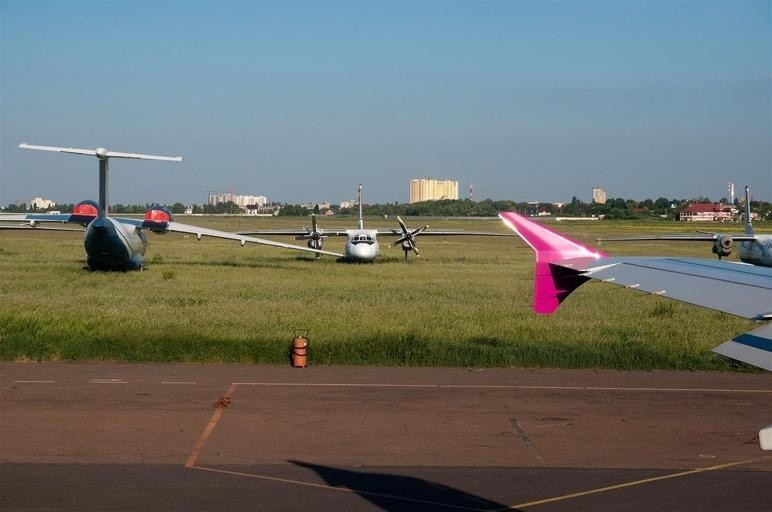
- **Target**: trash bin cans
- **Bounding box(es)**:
[292,335,309,368]
